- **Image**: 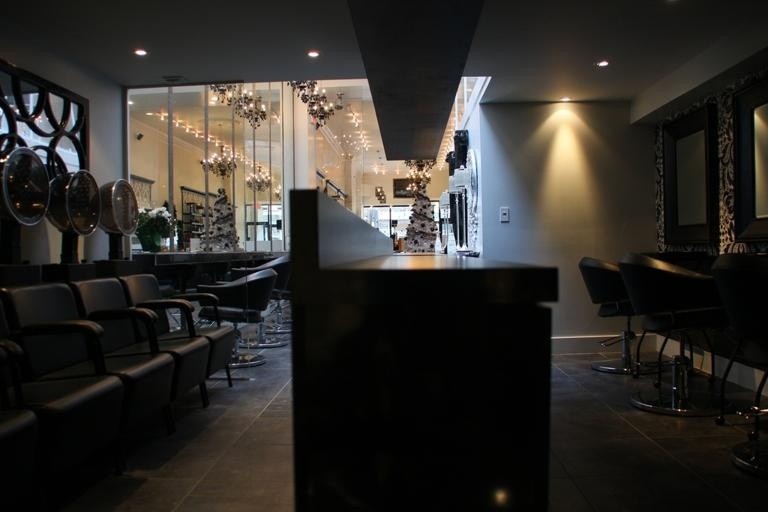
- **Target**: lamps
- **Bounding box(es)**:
[198,77,354,202]
[406,161,435,194]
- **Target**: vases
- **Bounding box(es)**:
[137,231,162,252]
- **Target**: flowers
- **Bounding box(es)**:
[134,204,176,240]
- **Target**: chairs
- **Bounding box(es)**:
[578,256,646,373]
[1,249,290,509]
[704,251,767,472]
[616,253,718,417]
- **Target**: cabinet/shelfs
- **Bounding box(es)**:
[287,180,563,511]
[182,201,214,248]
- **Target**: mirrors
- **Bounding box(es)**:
[731,80,768,244]
[661,105,720,245]
